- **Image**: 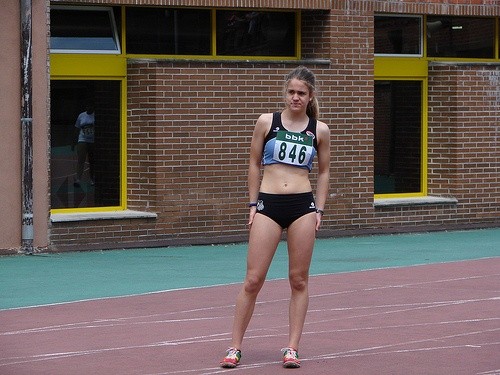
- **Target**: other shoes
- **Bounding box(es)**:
[73,179,80,186]
[90,180,95,185]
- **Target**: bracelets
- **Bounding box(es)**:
[248,203,257,207]
[315,209,323,216]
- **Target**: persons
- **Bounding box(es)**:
[218,65,331,367]
[72,102,94,185]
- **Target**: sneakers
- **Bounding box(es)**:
[219,348,241,367]
[281,347,301,368]
